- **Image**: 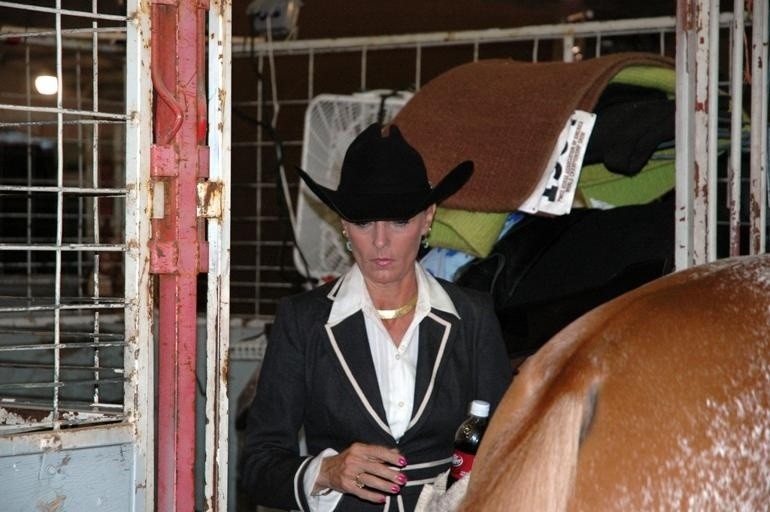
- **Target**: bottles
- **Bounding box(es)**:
[445,400,493,494]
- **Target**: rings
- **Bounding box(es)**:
[355,472,365,488]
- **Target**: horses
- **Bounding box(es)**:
[452,252,770,512]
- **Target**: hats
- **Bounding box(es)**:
[298,123,475,223]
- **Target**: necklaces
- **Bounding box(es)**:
[376,291,418,320]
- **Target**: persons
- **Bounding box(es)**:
[234,119,513,511]
